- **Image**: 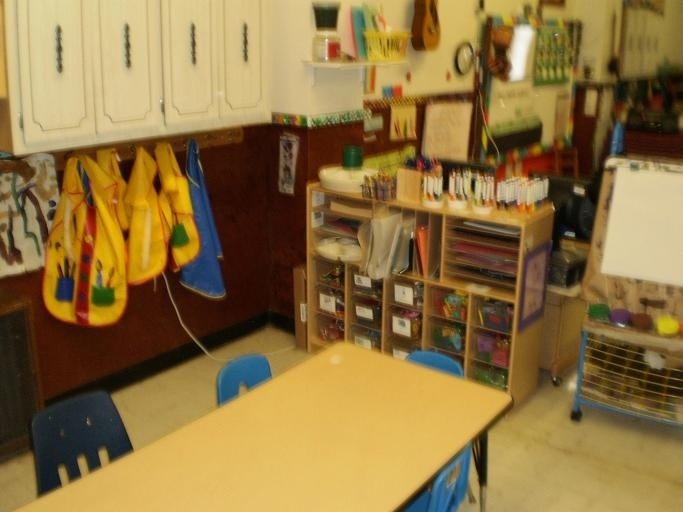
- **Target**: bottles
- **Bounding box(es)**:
[609,121,623,155]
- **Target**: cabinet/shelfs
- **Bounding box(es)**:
[2,2,166,147]
[301,161,558,415]
[161,2,272,138]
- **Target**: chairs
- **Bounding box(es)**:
[405,348,466,380]
[393,442,474,509]
[27,390,138,497]
[214,352,273,404]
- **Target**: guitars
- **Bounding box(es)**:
[411,0,441,51]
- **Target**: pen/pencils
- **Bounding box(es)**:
[360,167,398,201]
[415,155,550,214]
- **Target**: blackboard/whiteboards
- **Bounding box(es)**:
[0,295,47,458]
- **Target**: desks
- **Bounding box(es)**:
[2,339,512,509]
